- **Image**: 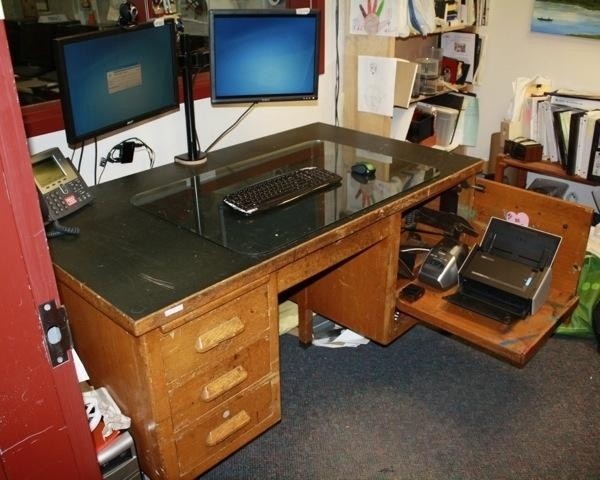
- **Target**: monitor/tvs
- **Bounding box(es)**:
[54,17,180,145]
[208,8,320,104]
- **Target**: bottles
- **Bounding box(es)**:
[415,57,439,95]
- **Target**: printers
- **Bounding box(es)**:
[440,215,563,326]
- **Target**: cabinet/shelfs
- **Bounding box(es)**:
[98,123,485,347]
[336,0,481,217]
[49,186,282,479]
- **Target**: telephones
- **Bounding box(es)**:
[30,147,94,225]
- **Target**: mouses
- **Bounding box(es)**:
[351,162,375,175]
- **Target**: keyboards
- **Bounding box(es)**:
[222,166,342,216]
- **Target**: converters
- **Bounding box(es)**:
[119,141,135,163]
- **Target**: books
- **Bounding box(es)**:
[391,0,600,185]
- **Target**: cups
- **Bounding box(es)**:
[424,45,446,91]
[429,106,461,147]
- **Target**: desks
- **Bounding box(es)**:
[495,149,598,187]
